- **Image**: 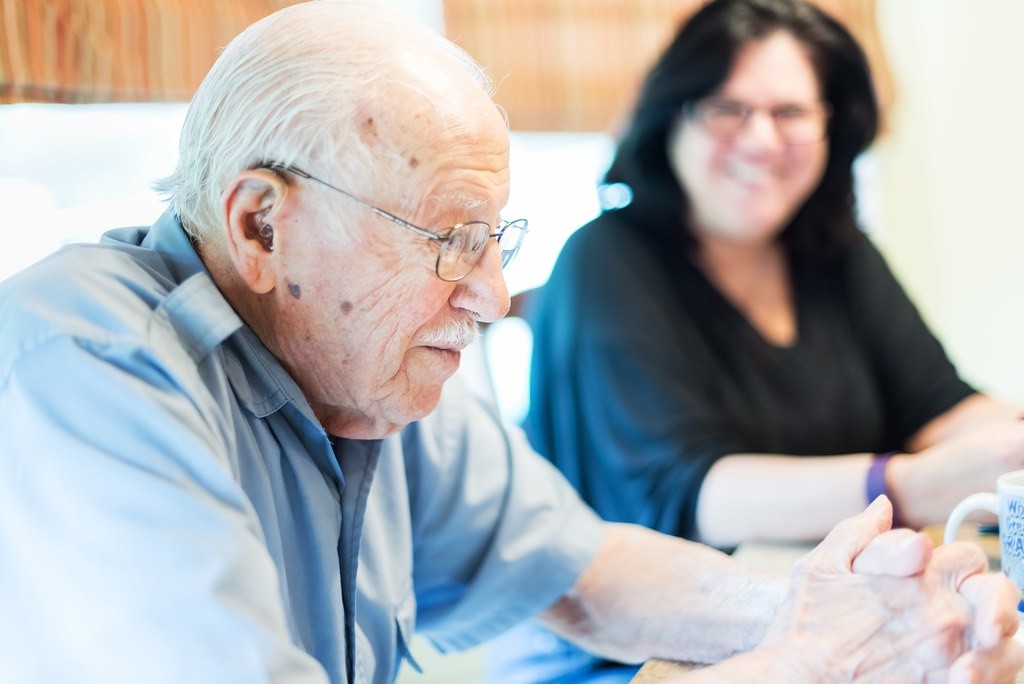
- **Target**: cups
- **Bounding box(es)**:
[943,470,1024,617]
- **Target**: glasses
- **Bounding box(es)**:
[250,160,529,283]
[689,95,834,145]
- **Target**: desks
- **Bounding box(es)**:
[628,521,1024,684]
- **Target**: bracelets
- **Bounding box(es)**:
[864,451,895,508]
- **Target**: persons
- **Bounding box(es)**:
[3,1,1024,684]
[481,2,1024,684]
